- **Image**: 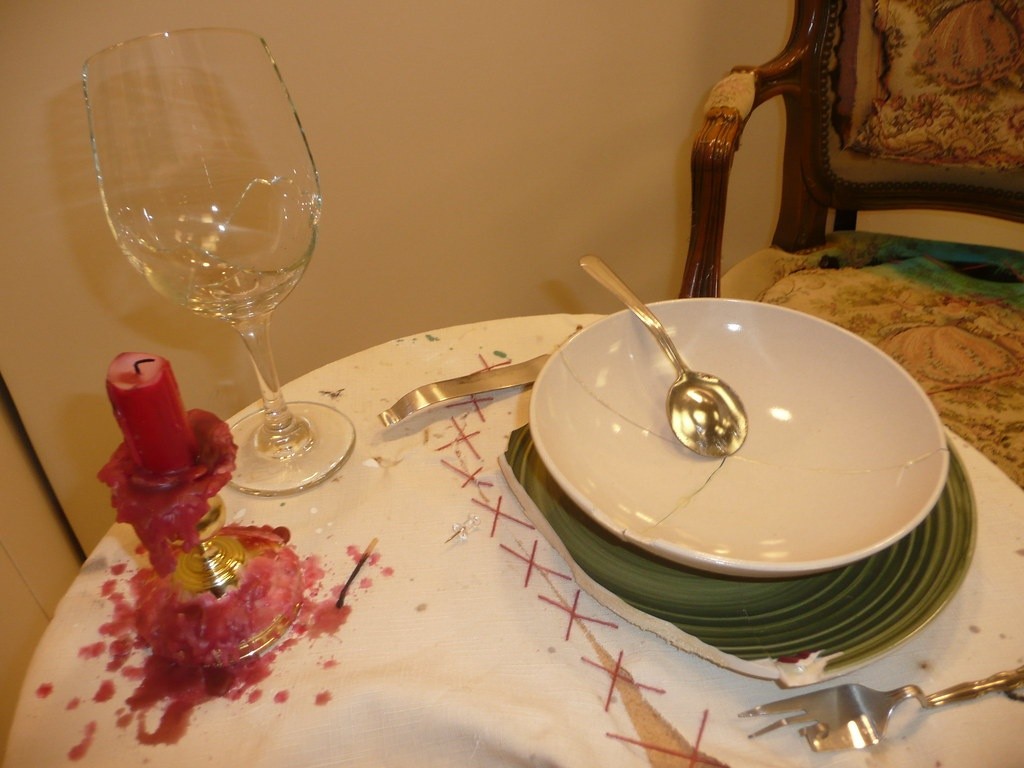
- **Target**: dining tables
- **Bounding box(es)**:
[0,312,1024,768]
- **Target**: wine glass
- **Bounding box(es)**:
[79,30,355,496]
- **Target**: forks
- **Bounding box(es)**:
[737,667,1024,753]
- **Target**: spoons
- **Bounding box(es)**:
[579,254,748,458]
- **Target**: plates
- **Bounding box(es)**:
[497,419,979,689]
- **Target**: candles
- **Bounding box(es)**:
[106,352,199,476]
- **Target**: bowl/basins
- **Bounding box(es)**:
[528,299,951,578]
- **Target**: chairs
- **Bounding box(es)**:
[677,0,1024,492]
